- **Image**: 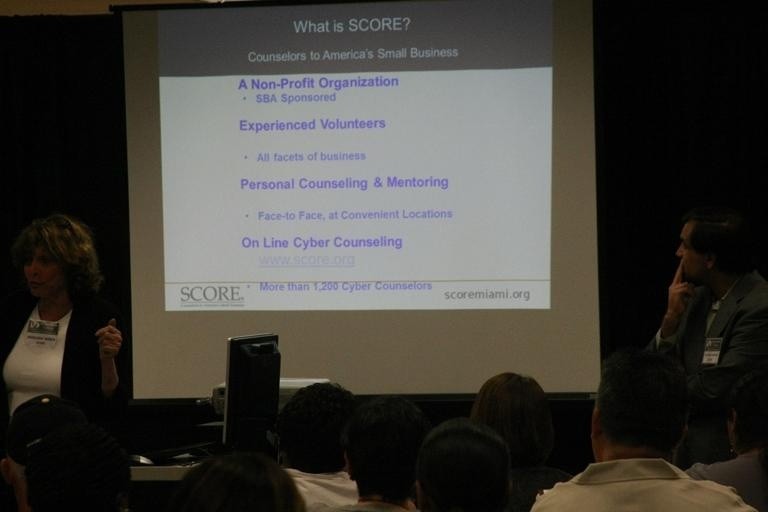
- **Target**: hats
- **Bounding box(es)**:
[7,394,90,466]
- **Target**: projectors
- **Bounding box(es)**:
[212,377,341,416]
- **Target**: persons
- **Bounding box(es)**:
[274,353,766,511]
[644,205,768,471]
[0,211,126,455]
[2,394,306,510]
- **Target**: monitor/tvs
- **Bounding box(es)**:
[223,333,281,449]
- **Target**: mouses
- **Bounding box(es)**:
[131,454,153,465]
